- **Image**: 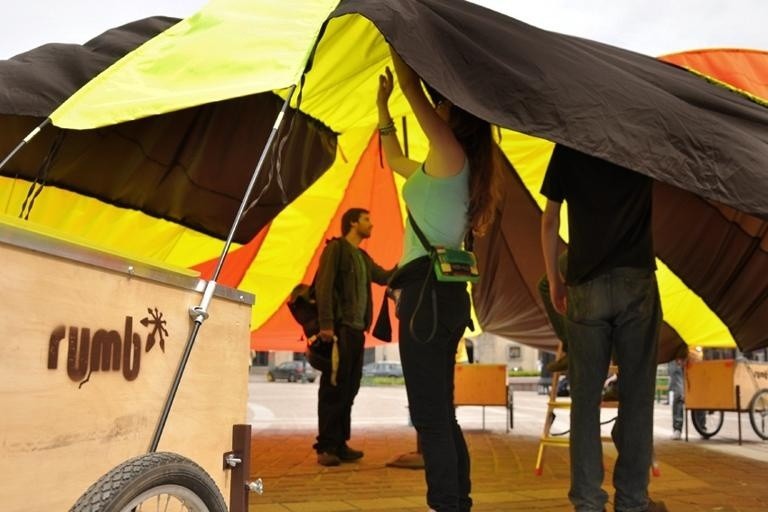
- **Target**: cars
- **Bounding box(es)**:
[267,359,316,383]
[362,362,404,378]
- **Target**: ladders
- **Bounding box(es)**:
[534,340,659,476]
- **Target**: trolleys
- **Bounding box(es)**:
[0,219,256,512]
[684,360,768,441]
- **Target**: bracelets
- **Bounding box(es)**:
[375,117,398,135]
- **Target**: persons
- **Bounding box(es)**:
[371,34,496,512]
[315,208,396,466]
[669,357,709,441]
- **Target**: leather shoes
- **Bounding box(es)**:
[317,445,363,465]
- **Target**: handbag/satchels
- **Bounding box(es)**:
[287,283,348,377]
[429,245,480,282]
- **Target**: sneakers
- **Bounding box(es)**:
[616,497,665,512]
[672,430,680,440]
